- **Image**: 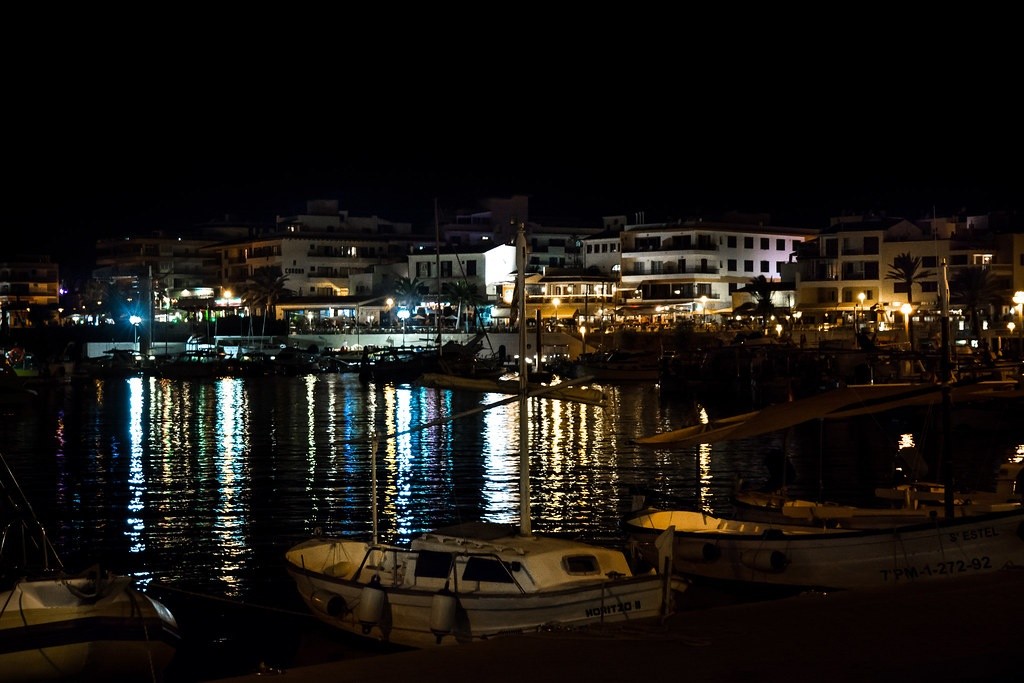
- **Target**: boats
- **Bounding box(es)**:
[0,566,182,683]
[610,254,1024,598]
[170,196,668,387]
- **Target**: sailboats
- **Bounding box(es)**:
[283,222,674,648]
